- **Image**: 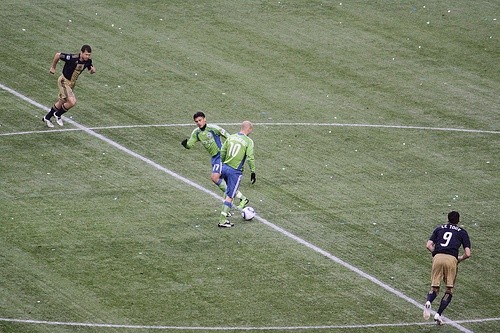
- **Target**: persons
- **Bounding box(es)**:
[181,112,250,216]
[217,121,256,227]
[423,211,471,325]
[42,45,96,128]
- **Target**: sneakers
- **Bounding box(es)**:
[42,116,54,128]
[226,210,232,217]
[422,300,432,319]
[238,196,251,208]
[433,313,444,325]
[53,112,64,127]
[217,219,235,228]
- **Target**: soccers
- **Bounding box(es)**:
[242,206,258,222]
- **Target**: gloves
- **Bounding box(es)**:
[181,139,188,147]
[250,173,256,184]
